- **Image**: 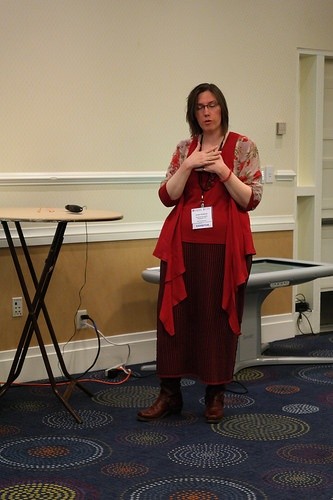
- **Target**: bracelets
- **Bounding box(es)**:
[220,171,232,182]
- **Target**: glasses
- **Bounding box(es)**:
[197,101,220,111]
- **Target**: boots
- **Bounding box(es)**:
[205,383,227,422]
[136,377,183,422]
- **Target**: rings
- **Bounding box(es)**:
[203,168,205,171]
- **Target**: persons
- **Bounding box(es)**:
[137,83,263,422]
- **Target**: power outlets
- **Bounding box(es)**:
[76,309,90,330]
[13,298,23,317]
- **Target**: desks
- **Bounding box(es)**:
[0,207,124,426]
